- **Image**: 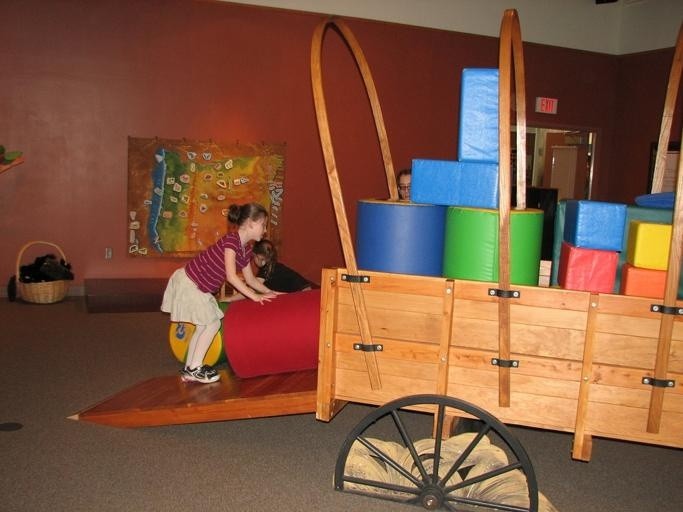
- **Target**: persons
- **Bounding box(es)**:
[159,200,291,384]
[396,168,412,201]
[219,238,312,303]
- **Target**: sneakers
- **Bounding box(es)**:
[179,365,221,384]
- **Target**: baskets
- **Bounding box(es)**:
[15,241,70,304]
